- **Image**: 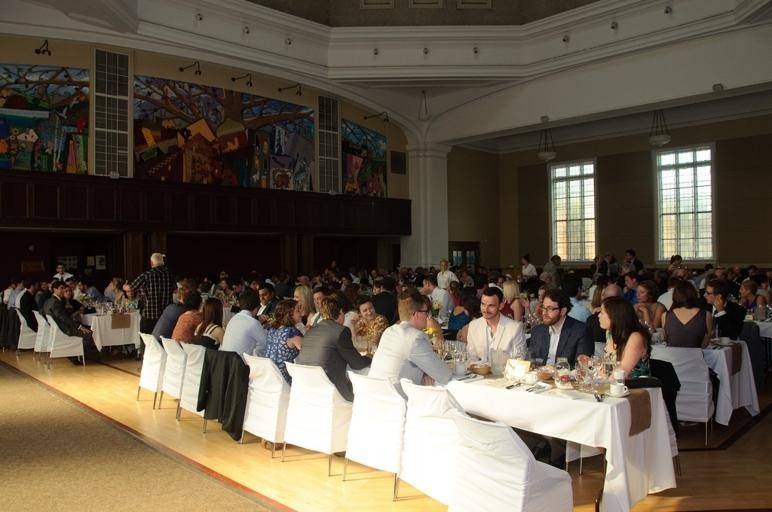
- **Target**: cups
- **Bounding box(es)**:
[720,337,730,344]
[610,384,628,394]
[491,346,521,376]
[651,332,660,343]
[437,340,467,375]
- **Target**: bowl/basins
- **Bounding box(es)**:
[537,370,554,380]
[470,364,491,375]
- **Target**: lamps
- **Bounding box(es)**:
[647,111,672,148]
[278,83,302,96]
[536,128,557,163]
[179,61,201,76]
[35,39,51,56]
[232,73,252,87]
[364,111,389,122]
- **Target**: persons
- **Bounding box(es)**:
[1,248,771,368]
[466,287,527,366]
[368,294,454,386]
[265,299,303,386]
[295,298,374,402]
[598,296,653,378]
[530,289,595,466]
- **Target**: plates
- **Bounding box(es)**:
[607,393,629,397]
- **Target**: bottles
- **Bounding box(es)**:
[639,320,654,344]
[613,361,625,384]
[555,355,613,394]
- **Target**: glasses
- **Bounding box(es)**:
[539,306,559,312]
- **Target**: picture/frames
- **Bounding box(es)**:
[456,0,494,10]
[359,0,398,9]
[408,0,443,9]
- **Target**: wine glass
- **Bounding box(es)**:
[522,313,541,330]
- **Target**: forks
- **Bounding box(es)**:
[458,375,478,380]
